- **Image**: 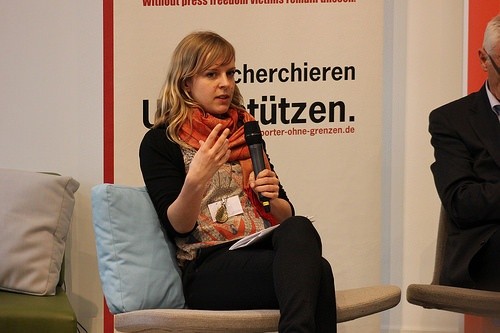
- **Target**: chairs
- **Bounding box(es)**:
[405,200,500,333]
[0,165,83,333]
[91,184,402,333]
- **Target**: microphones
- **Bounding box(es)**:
[243,120,272,212]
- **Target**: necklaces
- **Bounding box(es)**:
[216,163,233,223]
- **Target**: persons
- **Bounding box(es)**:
[139,31,337,333]
[428,14,500,291]
